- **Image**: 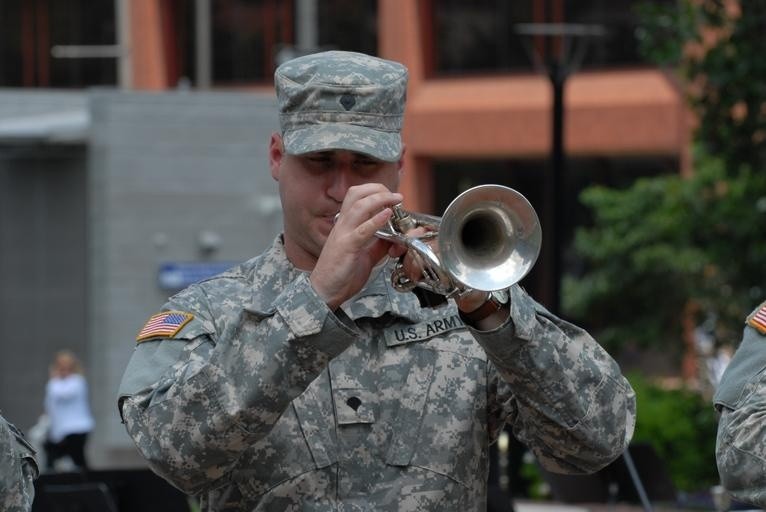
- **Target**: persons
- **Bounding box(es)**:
[41,349,95,471]
[710,298,766,511]
[116,49,637,512]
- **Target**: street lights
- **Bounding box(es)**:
[513,17,604,322]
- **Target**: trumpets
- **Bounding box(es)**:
[334,184,542,300]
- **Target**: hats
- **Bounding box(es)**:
[273,48,410,163]
